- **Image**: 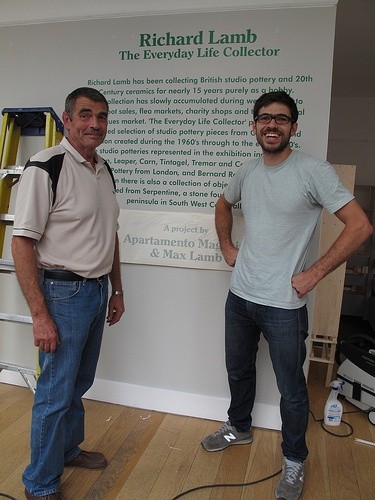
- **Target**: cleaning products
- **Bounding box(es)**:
[324,380,345,426]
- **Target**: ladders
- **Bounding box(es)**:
[0,105,64,401]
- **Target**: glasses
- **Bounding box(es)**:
[255,113,294,125]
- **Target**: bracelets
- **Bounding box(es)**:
[112,289,123,296]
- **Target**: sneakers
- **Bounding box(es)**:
[200,420,254,452]
[274,456,305,500]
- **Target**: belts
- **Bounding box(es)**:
[44,269,105,282]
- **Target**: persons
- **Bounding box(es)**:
[11,87,126,500]
[198,89,373,500]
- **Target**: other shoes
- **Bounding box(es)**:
[64,450,108,470]
[24,487,66,500]
